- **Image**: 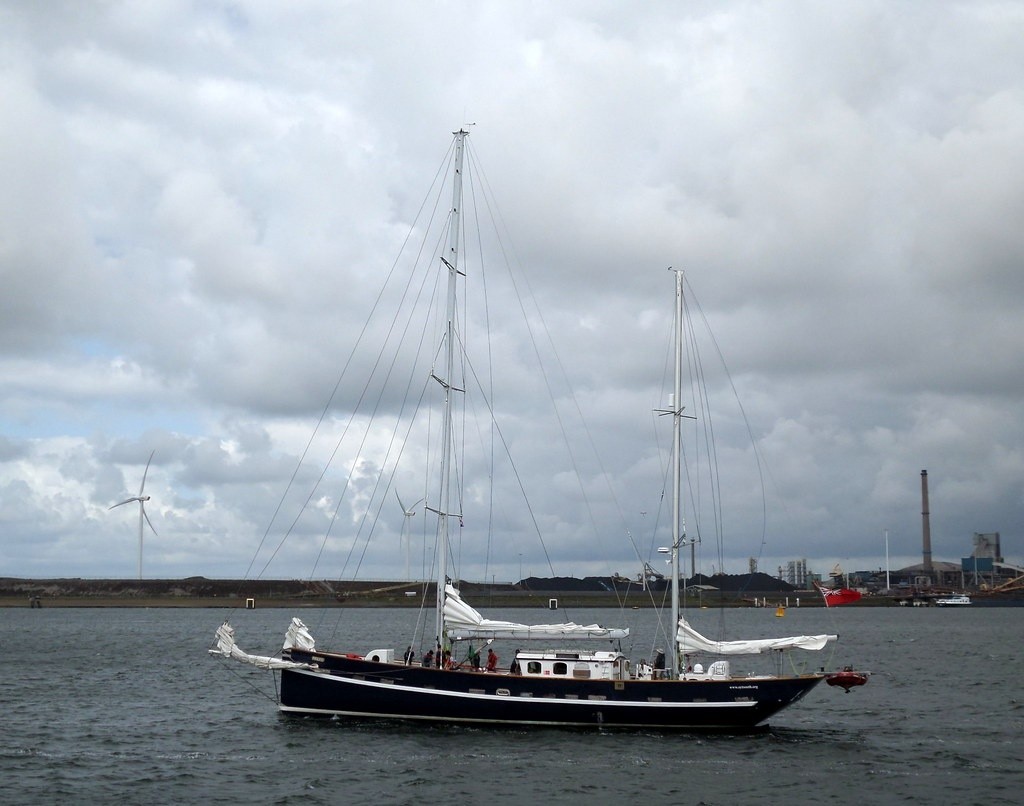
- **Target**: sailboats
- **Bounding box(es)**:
[206,126,873,729]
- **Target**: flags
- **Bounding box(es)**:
[818,586,862,607]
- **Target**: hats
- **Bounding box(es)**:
[657,649,665,654]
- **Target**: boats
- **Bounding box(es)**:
[934,595,973,607]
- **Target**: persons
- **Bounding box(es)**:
[510,649,521,674]
[653,649,665,680]
[423,644,451,669]
[684,654,692,672]
[404,646,415,666]
[469,645,480,672]
[640,659,646,669]
[486,649,498,673]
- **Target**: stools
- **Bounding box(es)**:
[653,669,667,677]
